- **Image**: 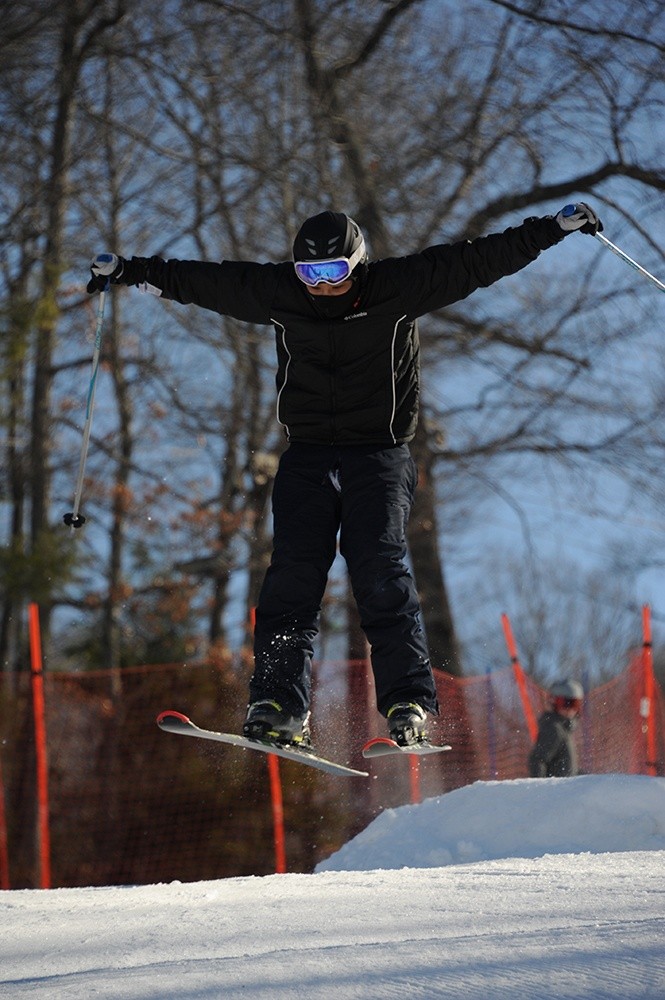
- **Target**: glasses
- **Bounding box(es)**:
[292,237,365,288]
[554,698,581,711]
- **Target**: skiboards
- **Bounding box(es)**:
[155,709,453,780]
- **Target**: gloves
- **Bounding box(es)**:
[553,202,604,235]
[85,252,125,293]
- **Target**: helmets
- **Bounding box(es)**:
[295,212,362,281]
[546,679,584,701]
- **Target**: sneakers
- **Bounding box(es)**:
[385,703,431,749]
[240,698,313,749]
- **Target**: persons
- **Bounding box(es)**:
[525,676,588,781]
[83,198,608,748]
